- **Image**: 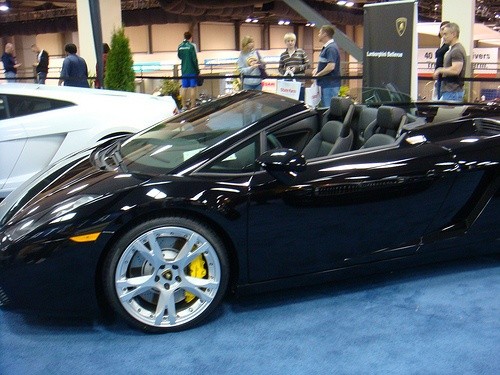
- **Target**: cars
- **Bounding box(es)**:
[0,82,181,195]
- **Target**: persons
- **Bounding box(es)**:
[30,43,49,84]
[277,32,310,101]
[95,43,110,89]
[311,24,342,107]
[434,21,467,101]
[177,32,200,112]
[237,36,263,127]
[58,43,90,89]
[1,43,22,83]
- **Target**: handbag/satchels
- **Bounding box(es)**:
[257,51,268,80]
[304,77,322,108]
[277,69,300,101]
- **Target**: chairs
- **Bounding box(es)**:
[300,97,356,160]
[359,105,407,150]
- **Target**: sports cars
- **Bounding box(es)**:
[0,88,500,336]
